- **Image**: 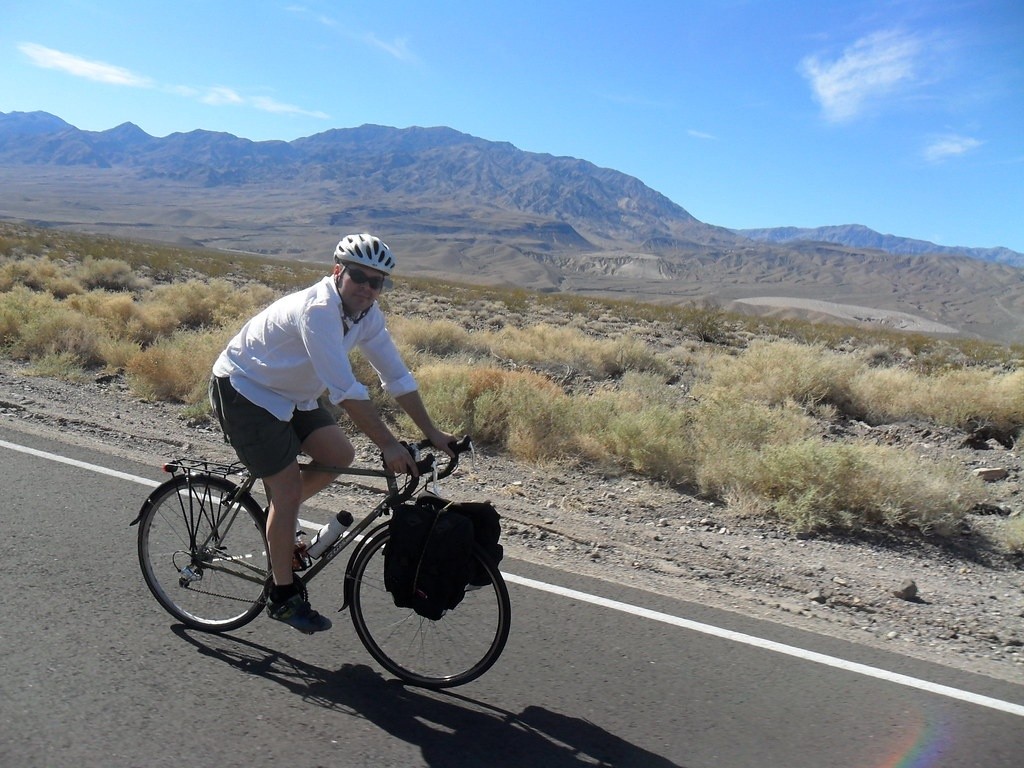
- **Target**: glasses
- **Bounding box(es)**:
[344,268,383,290]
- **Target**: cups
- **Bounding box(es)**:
[306,510,354,559]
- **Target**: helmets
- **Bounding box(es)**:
[333,233,397,277]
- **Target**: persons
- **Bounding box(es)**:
[207,233,460,635]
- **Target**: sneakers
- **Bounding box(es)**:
[265,594,333,634]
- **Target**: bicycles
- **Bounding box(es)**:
[128,434,512,689]
